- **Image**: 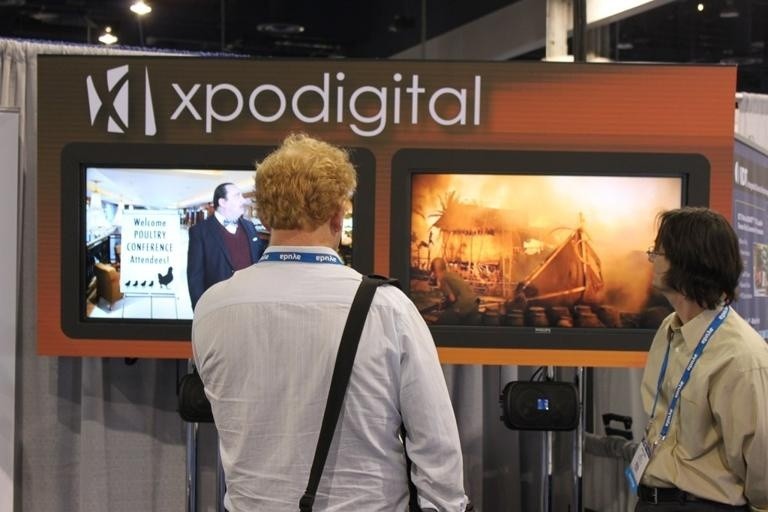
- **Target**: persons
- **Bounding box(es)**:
[191,133,469,512]
[754,246,768,289]
[634,207,768,511]
[425,255,482,325]
[184,182,266,312]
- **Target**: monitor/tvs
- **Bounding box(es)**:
[391,148,709,352]
[59,143,375,341]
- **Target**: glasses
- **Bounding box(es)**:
[648,247,666,262]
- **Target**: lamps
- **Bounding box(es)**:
[98,26,118,44]
[130,0,152,15]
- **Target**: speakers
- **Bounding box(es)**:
[499,379,578,431]
[179,375,214,422]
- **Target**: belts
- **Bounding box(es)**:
[636,486,699,504]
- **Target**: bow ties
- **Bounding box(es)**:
[224,219,238,226]
